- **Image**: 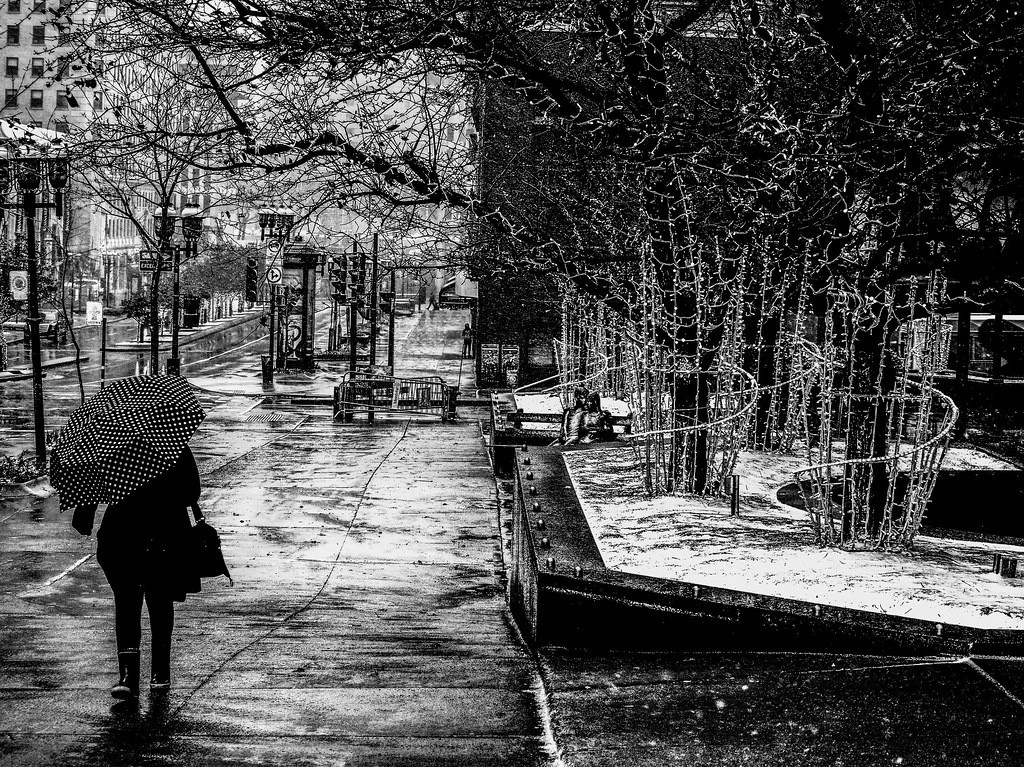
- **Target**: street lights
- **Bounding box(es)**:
[1,142,68,481]
[153,204,207,377]
[258,207,295,393]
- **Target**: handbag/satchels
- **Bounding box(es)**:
[192,502,234,587]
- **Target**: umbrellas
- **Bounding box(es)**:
[49,373,208,515]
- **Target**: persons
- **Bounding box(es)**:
[71,444,202,701]
[546,386,613,447]
[425,290,439,309]
[462,323,473,359]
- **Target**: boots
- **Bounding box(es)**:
[149,640,172,690]
[109,647,141,699]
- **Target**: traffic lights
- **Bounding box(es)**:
[330,255,346,305]
[182,297,200,329]
[377,292,394,314]
[245,257,258,301]
[349,256,366,307]
